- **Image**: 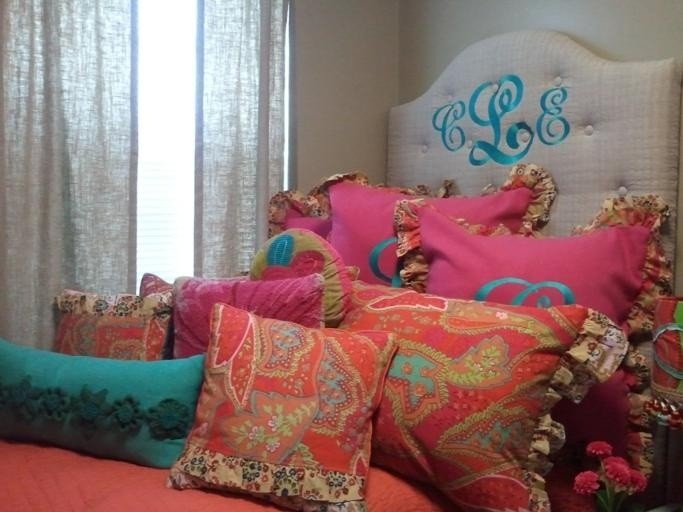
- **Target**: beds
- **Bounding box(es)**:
[1,31,682,512]
